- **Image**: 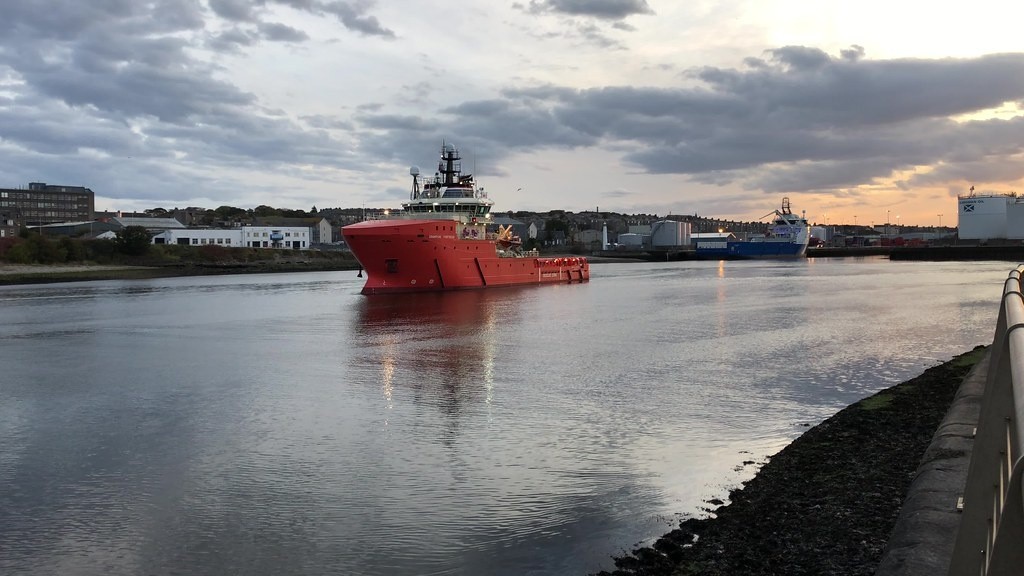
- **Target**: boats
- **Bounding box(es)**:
[695,198,812,258]
[340,139,589,295]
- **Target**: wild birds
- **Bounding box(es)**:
[517,188,521,191]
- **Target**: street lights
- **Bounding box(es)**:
[938,214,943,239]
[854,216,857,225]
[888,211,890,224]
